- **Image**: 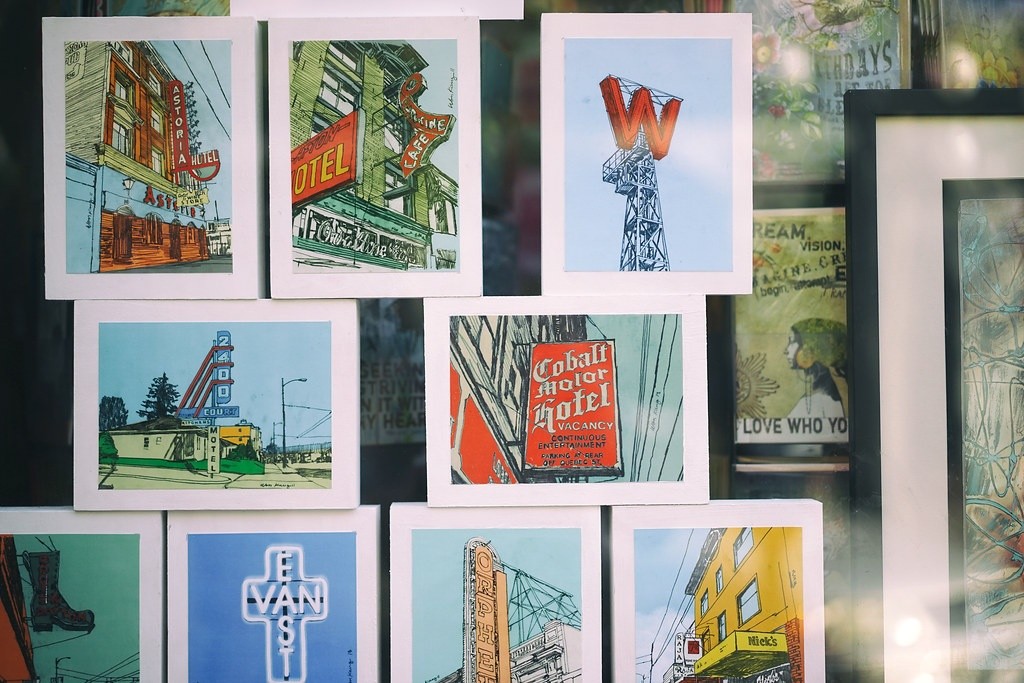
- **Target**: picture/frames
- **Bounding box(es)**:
[729,463,857,683]
[722,183,849,458]
[848,85,1024,683]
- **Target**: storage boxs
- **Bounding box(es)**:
[0,0,915,682]
